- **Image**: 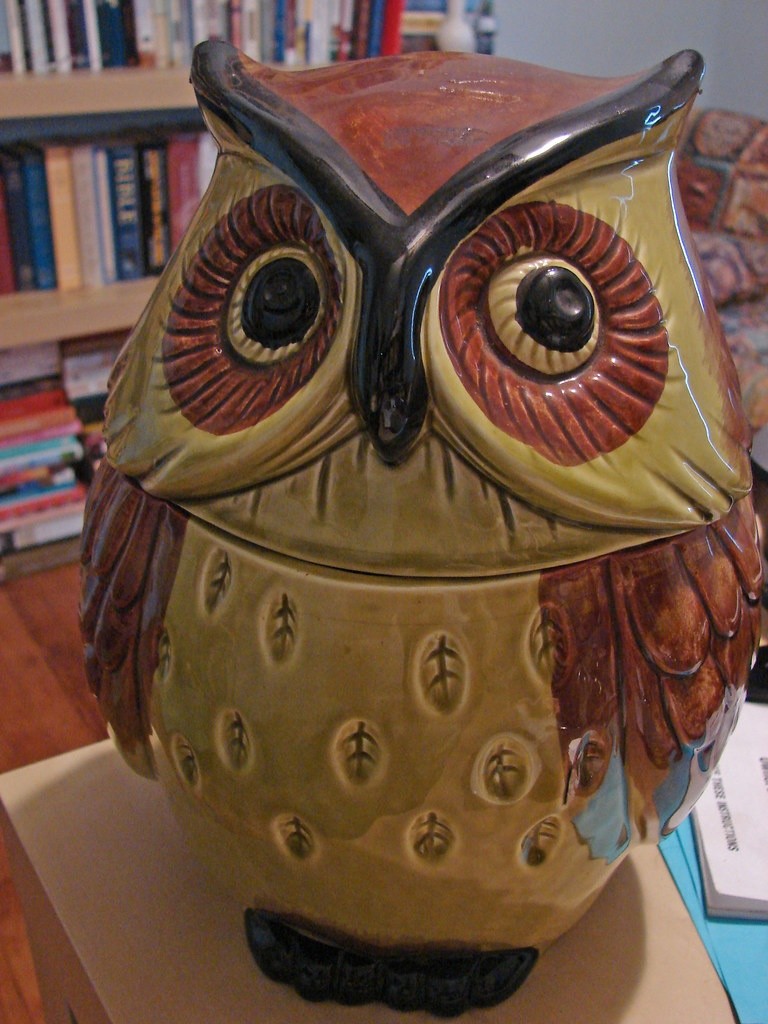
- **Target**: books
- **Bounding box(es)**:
[689,701,767,922]
[0,0,403,553]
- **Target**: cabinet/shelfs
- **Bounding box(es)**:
[0,65,363,594]
